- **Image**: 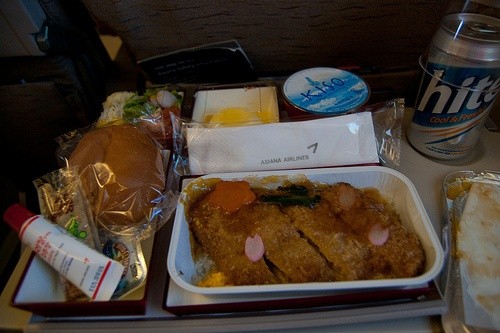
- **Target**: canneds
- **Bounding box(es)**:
[407,13,500,161]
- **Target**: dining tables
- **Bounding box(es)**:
[0,107,500,332]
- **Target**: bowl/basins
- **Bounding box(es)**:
[167,166,446,294]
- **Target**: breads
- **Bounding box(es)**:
[66,123,166,226]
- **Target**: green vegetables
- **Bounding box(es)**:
[123,85,182,124]
